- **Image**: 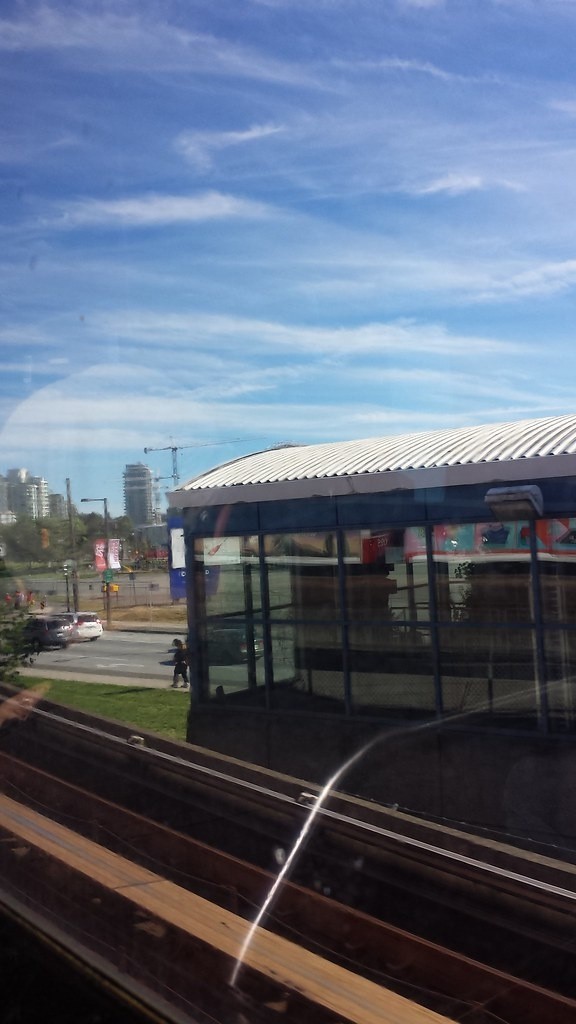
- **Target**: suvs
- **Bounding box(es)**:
[48,611,103,643]
[22,617,74,653]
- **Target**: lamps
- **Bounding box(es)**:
[483,484,544,522]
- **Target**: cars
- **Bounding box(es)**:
[207,628,263,665]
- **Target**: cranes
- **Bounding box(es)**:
[144,438,248,485]
[116,468,171,526]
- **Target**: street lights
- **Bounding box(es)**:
[484,485,548,731]
[80,497,112,626]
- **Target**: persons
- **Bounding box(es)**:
[27,592,35,609]
[4,593,12,604]
[40,595,47,609]
[170,639,189,688]
[14,589,24,610]
